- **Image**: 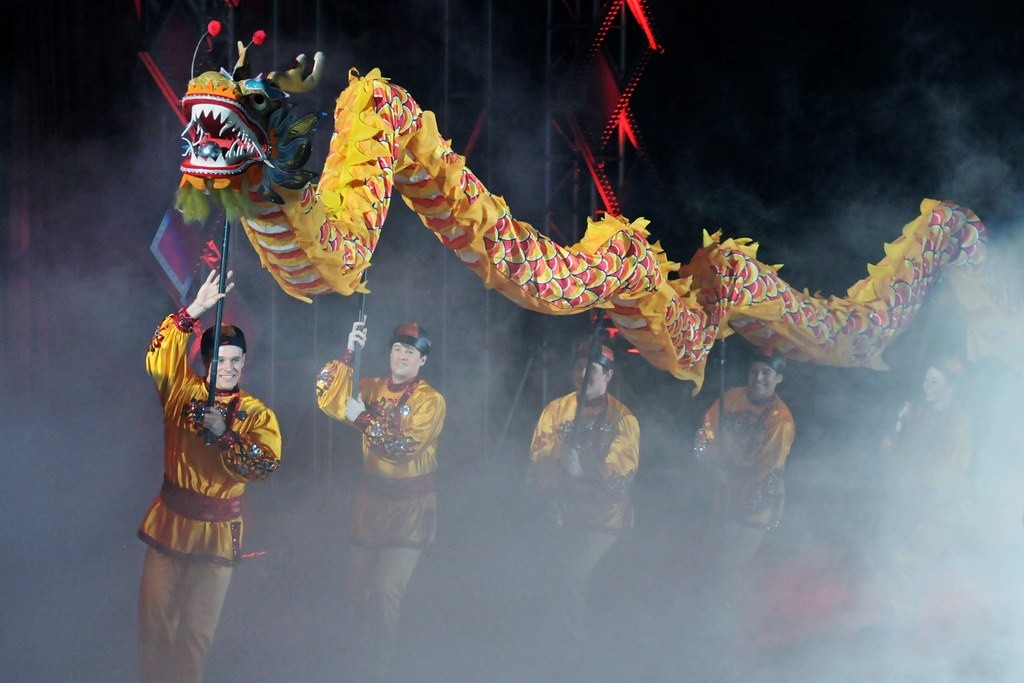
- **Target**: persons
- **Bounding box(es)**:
[316,314,447,639]
[528,342,641,576]
[138,270,283,682]
[696,347,795,555]
[882,355,972,508]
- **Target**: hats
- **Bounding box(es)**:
[200,323,246,355]
[577,337,614,369]
[750,347,786,376]
[933,356,964,384]
[390,322,431,358]
